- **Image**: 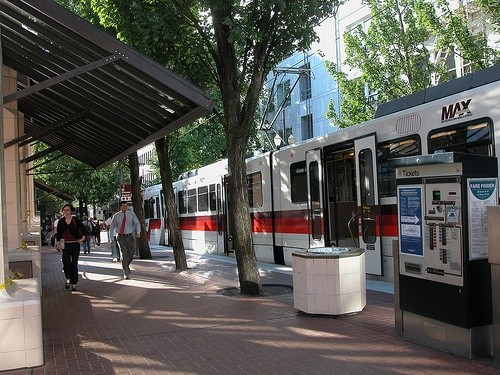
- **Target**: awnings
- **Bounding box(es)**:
[0,0,215,172]
[35,178,74,202]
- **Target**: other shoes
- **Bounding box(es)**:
[88,250,90,253]
[71,286,77,291]
[113,257,117,262]
[123,268,131,279]
[84,250,86,254]
[65,282,70,289]
[98,246,100,247]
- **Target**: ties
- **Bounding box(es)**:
[120,213,127,236]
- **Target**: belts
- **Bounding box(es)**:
[118,233,132,236]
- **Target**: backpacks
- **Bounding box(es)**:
[84,222,90,234]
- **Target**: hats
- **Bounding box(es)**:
[83,216,87,219]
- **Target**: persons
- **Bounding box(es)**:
[50,210,120,259]
[56,204,86,291]
[109,201,141,279]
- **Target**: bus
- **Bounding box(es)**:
[103,185,133,218]
[141,62,500,284]
